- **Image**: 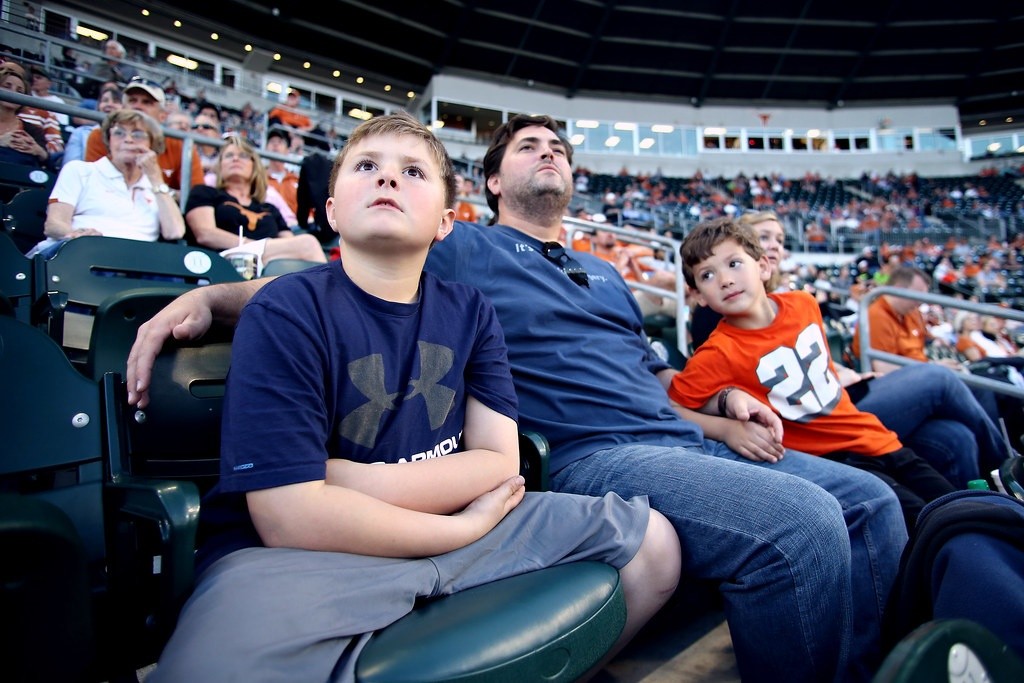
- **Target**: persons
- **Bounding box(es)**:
[126,113,909,683]
[137,110,682,683]
[686,210,1012,493]
[667,217,961,541]
[0,36,1024,428]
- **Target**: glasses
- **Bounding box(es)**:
[191,123,218,132]
[542,241,590,290]
[130,78,163,88]
[110,127,149,141]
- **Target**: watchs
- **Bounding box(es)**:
[152,183,169,194]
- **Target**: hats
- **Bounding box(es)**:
[121,76,165,108]
[30,67,52,82]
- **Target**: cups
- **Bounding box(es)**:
[224,249,258,281]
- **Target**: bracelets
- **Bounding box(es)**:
[718,386,736,418]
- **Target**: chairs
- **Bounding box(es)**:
[0,95,1024,683]
